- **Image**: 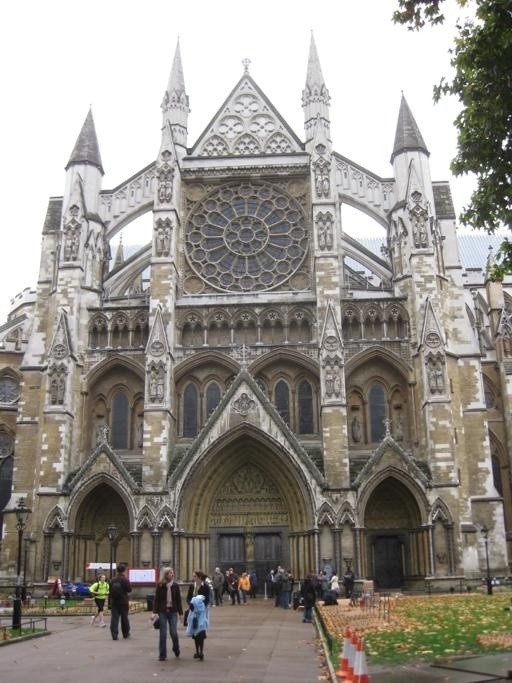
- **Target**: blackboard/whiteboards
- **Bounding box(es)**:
[129,566,158,586]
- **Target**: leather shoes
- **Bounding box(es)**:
[194,653,199,658]
[173,647,180,656]
[199,653,204,660]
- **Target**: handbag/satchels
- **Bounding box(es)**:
[183,609,190,626]
[148,613,162,629]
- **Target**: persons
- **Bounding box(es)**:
[299,574,317,624]
[204,564,355,611]
[107,564,133,640]
[186,569,210,661]
[151,566,184,661]
[87,574,111,629]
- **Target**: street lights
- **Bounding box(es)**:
[22,532,28,601]
[481,526,492,594]
[13,497,33,629]
[107,524,117,581]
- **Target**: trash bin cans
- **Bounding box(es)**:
[146,594,155,611]
[293,591,304,610]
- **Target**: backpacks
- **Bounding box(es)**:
[111,576,125,600]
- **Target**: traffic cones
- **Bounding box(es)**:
[335,625,370,683]
